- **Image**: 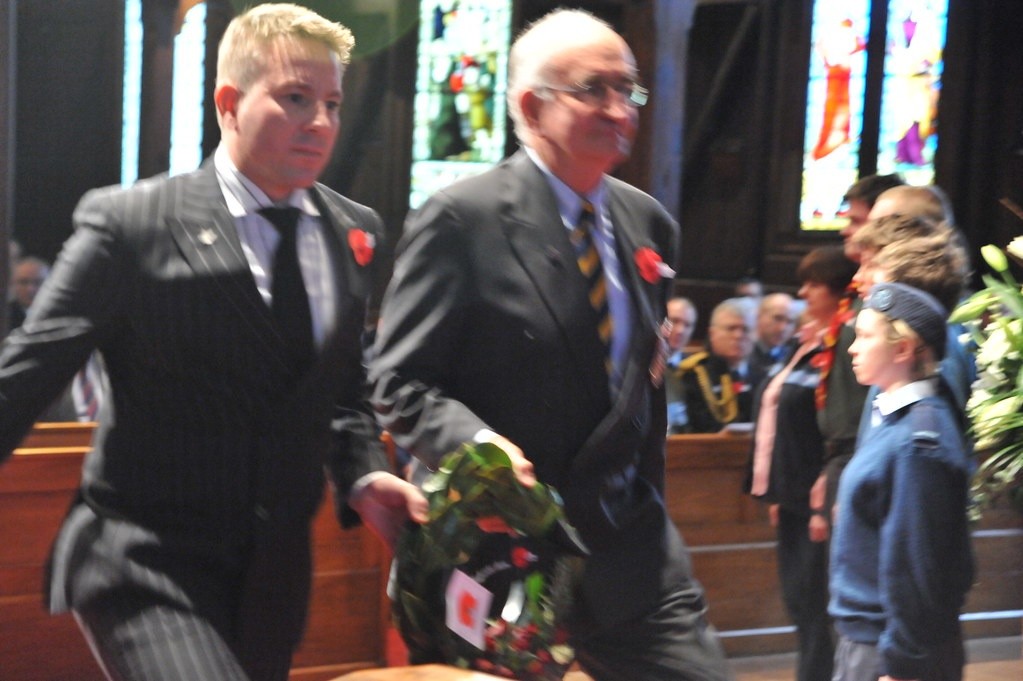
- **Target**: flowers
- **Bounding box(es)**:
[634,247,665,287]
[346,228,376,268]
[944,234,1023,521]
[384,440,592,681]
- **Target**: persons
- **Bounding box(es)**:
[366,7,730,681]
[664,173,984,680]
[0,3,433,681]
[0,242,112,428]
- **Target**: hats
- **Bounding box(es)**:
[859,283,949,361]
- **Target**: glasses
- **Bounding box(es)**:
[531,79,649,107]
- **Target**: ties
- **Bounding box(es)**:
[568,198,613,382]
[731,371,742,382]
[255,208,315,379]
